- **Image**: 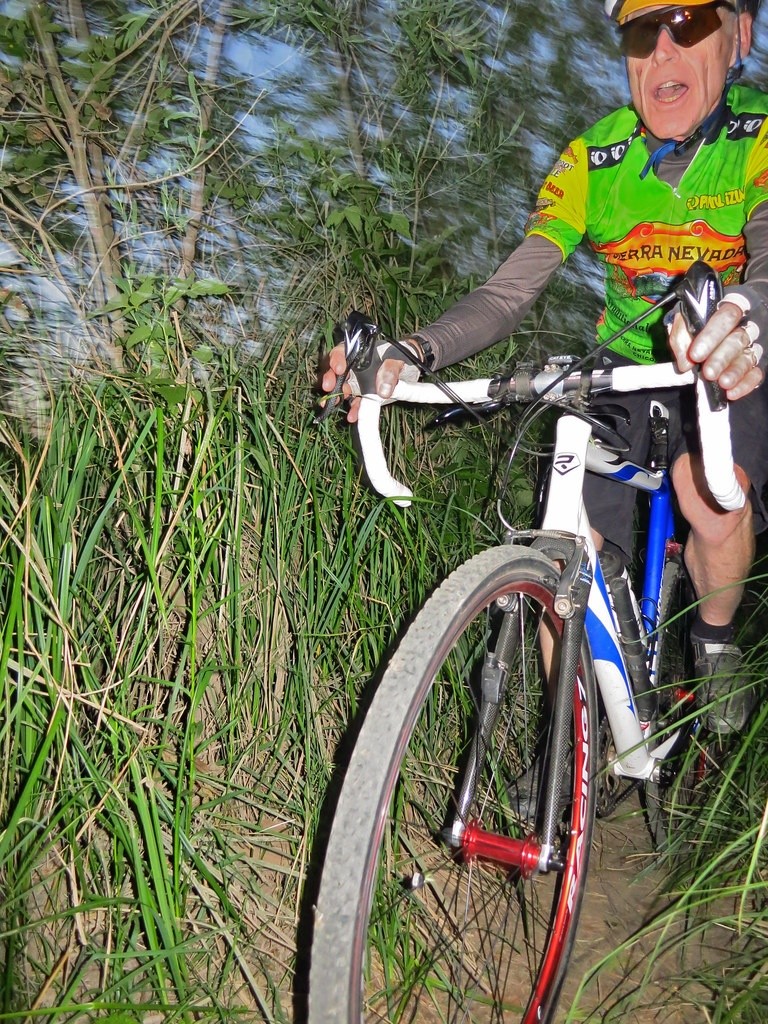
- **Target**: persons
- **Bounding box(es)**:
[315,0,768,818]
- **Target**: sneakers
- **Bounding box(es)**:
[507,728,573,817]
[689,631,753,734]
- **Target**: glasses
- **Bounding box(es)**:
[615,0,736,59]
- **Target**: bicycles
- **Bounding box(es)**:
[306,254,748,1024]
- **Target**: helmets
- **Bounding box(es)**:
[605,0,740,25]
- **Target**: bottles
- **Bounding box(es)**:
[596,550,648,649]
